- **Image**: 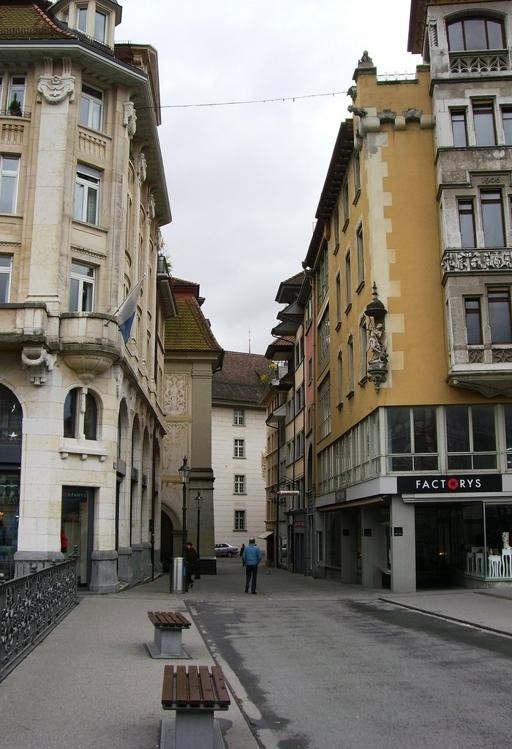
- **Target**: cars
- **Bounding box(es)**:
[215,542,238,557]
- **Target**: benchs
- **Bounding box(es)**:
[161,666,231,749]
[145,611,191,658]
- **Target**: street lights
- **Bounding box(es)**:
[179,455,192,558]
[194,490,203,577]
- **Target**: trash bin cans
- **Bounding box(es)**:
[170,557,185,594]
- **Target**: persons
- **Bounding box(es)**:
[242,537,262,594]
[240,544,245,567]
[369,323,383,361]
[183,541,197,588]
